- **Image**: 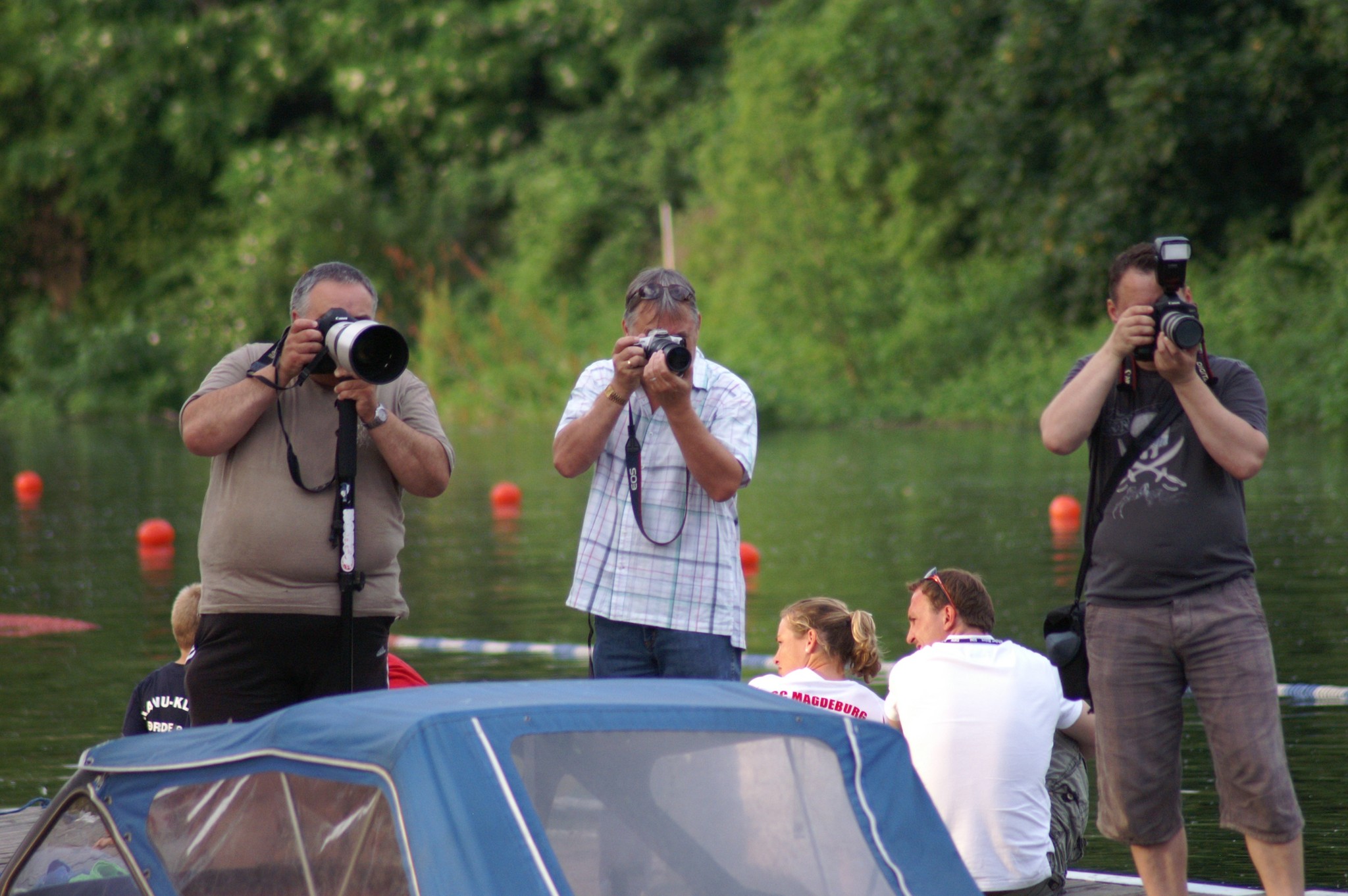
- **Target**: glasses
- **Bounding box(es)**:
[627,284,697,302]
[924,565,959,617]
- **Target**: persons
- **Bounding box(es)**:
[883,566,1095,896]
[1040,242,1307,896]
[387,652,429,689]
[553,267,757,681]
[121,582,202,739]
[739,597,886,895]
[179,262,456,729]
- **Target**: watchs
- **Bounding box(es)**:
[604,384,628,407]
[363,402,387,429]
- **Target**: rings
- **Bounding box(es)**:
[649,377,656,382]
[627,360,633,368]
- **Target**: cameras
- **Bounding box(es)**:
[1132,236,1204,363]
[632,329,692,372]
[298,307,409,386]
